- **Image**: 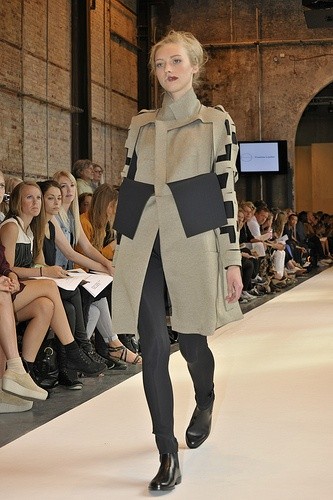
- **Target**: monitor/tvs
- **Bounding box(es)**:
[235,140,288,177]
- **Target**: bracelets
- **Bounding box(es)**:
[40,267,42,276]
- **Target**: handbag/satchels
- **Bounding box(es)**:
[30,339,60,387]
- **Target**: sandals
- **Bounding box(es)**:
[107,345,143,365]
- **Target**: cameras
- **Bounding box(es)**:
[4,193,10,202]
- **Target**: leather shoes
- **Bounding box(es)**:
[185,397,213,449]
[150,448,181,491]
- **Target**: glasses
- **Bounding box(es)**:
[95,170,103,174]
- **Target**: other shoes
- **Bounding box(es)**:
[238,252,333,303]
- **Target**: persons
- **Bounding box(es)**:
[111,31,244,490]
[236,200,333,304]
[0,159,142,413]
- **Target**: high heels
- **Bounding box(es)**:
[0,390,33,413]
[1,369,48,400]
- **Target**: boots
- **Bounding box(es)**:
[58,345,83,390]
[65,340,106,377]
[95,342,127,370]
[80,341,116,370]
